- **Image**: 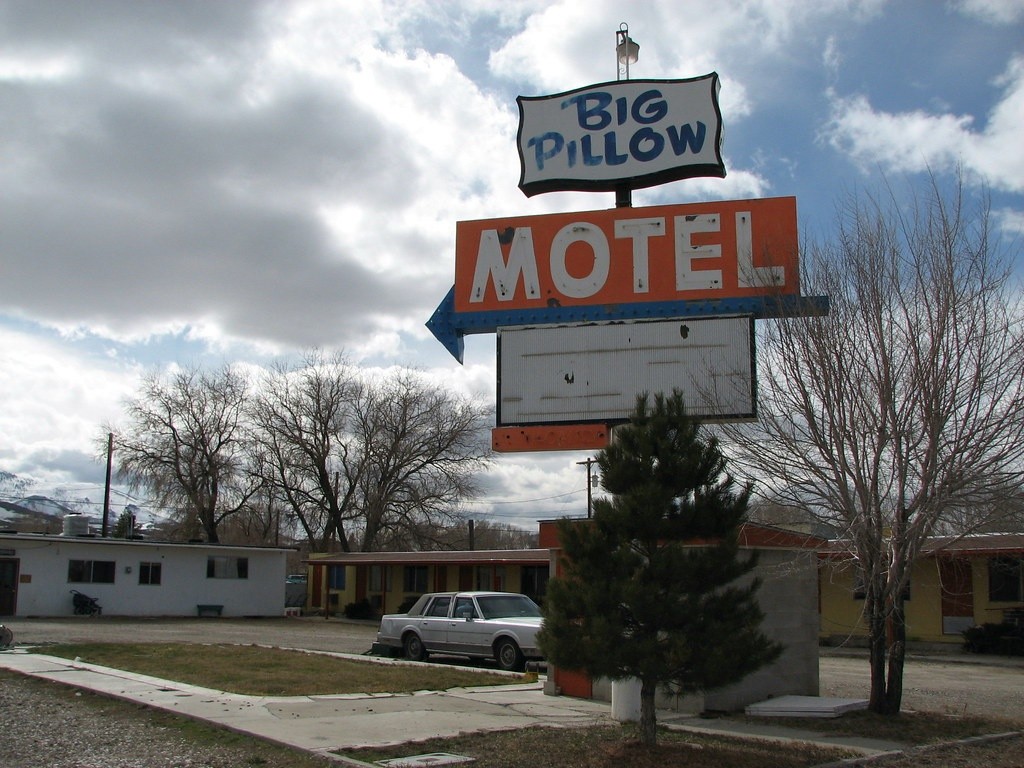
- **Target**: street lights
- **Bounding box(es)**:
[615,21,640,208]
[576,458,599,517]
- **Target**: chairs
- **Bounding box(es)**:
[456,604,477,618]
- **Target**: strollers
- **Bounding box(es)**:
[70,590,103,615]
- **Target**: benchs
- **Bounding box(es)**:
[195,604,223,617]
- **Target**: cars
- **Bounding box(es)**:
[377,591,548,671]
